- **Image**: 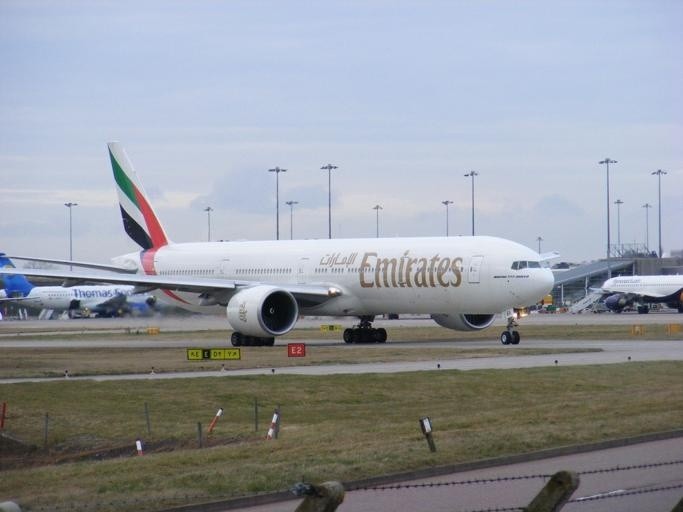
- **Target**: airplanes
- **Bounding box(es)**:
[587,273,682,314]
[1,252,168,319]
[1,234,555,348]
